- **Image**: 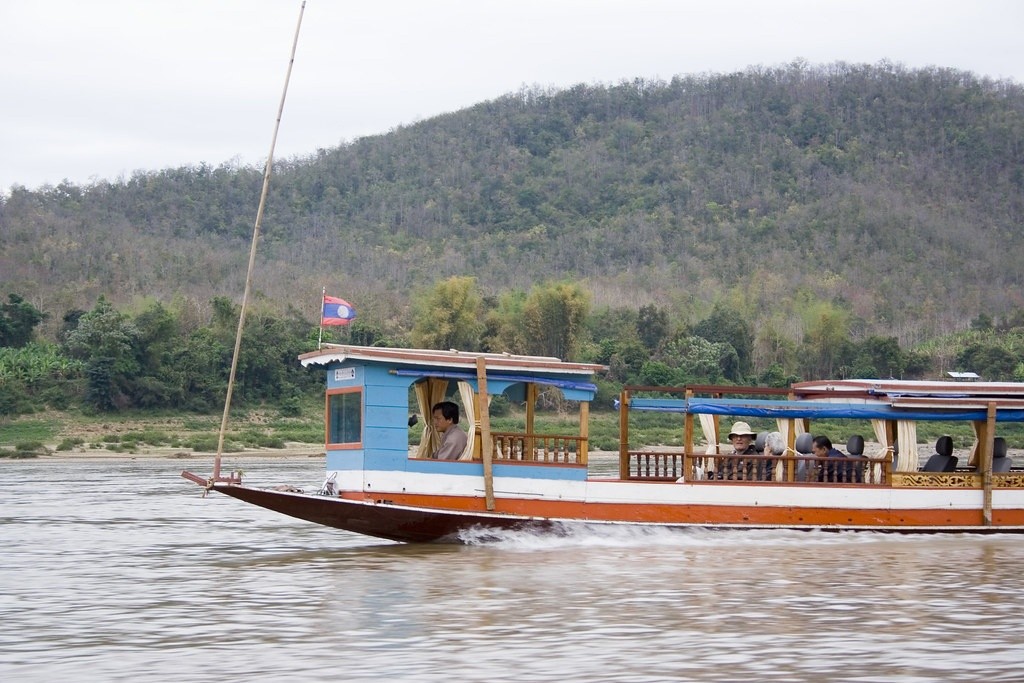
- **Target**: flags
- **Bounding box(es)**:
[323,296,356,325]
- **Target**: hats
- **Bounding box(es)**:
[729,421,757,441]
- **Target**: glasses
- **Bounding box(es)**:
[432,416,446,421]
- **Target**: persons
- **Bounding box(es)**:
[718,420,759,479]
[747,432,785,481]
[432,402,468,459]
[812,436,860,483]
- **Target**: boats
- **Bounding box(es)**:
[179,1,1023,542]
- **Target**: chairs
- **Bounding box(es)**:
[752,431,1014,482]
